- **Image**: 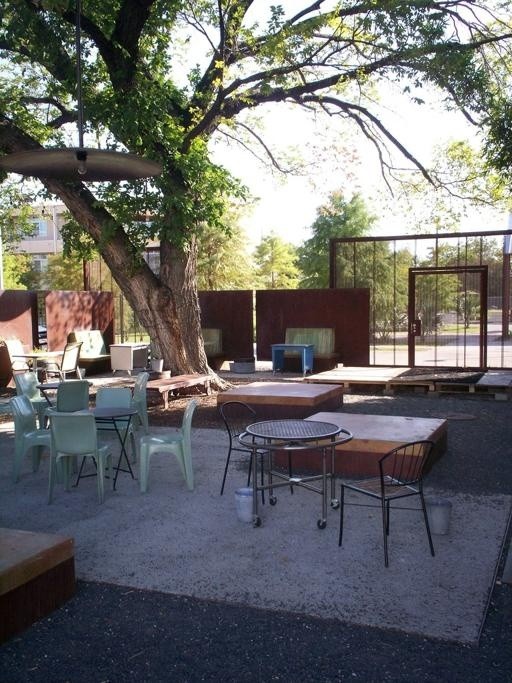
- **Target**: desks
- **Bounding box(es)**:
[237,418,352,536]
[268,342,312,375]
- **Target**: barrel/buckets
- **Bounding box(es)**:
[235,485,258,522]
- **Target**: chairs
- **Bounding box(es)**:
[218,401,294,504]
[337,439,434,567]
[3,335,198,506]
